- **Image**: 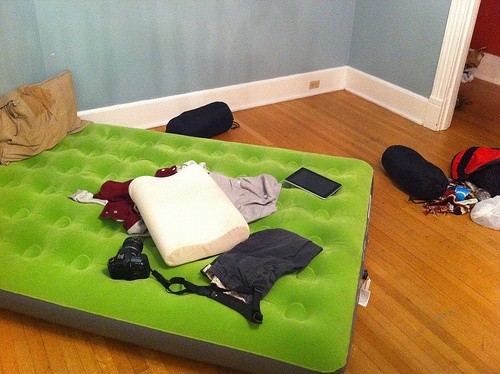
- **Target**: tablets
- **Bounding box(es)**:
[284,167,342,200]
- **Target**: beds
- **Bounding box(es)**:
[0,122,375,374]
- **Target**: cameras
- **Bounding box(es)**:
[107,236,150,281]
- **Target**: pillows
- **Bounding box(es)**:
[0,69,94,166]
[128,159,251,267]
